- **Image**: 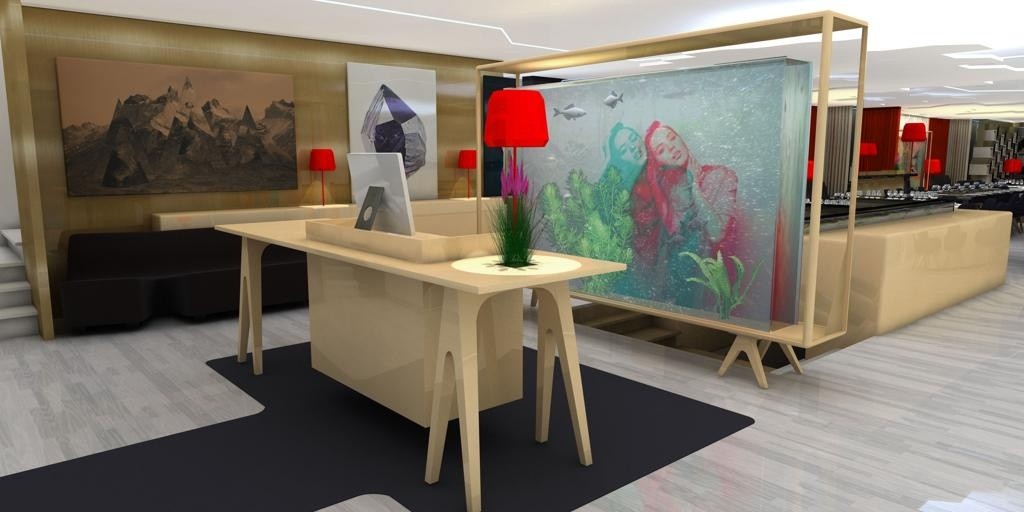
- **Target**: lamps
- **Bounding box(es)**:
[309,149,337,206]
[923,159,941,185]
[901,123,926,174]
[458,150,476,198]
[484,91,548,227]
[1004,160,1022,179]
[860,143,877,170]
[807,160,814,180]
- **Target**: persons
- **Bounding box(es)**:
[598,122,647,238]
[628,121,747,312]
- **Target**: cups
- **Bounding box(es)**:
[805,179,1023,206]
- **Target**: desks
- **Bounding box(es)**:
[215,219,627,511]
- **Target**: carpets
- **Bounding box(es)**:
[0,340,755,512]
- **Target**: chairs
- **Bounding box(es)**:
[61,228,309,333]
